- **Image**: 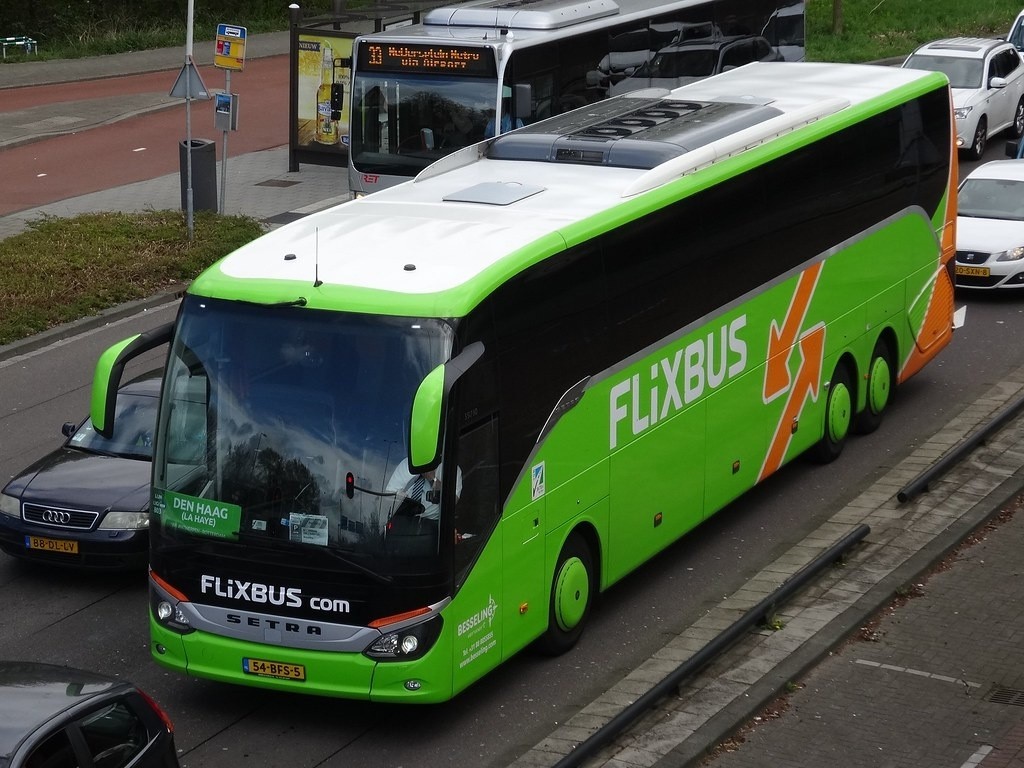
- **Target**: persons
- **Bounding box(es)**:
[484,103,524,139]
[383,457,462,520]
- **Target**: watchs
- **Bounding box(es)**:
[430,477,437,484]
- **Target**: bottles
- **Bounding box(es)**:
[314,49,340,145]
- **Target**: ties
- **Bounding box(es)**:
[411,475,424,501]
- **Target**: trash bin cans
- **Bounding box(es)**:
[179,138,219,216]
